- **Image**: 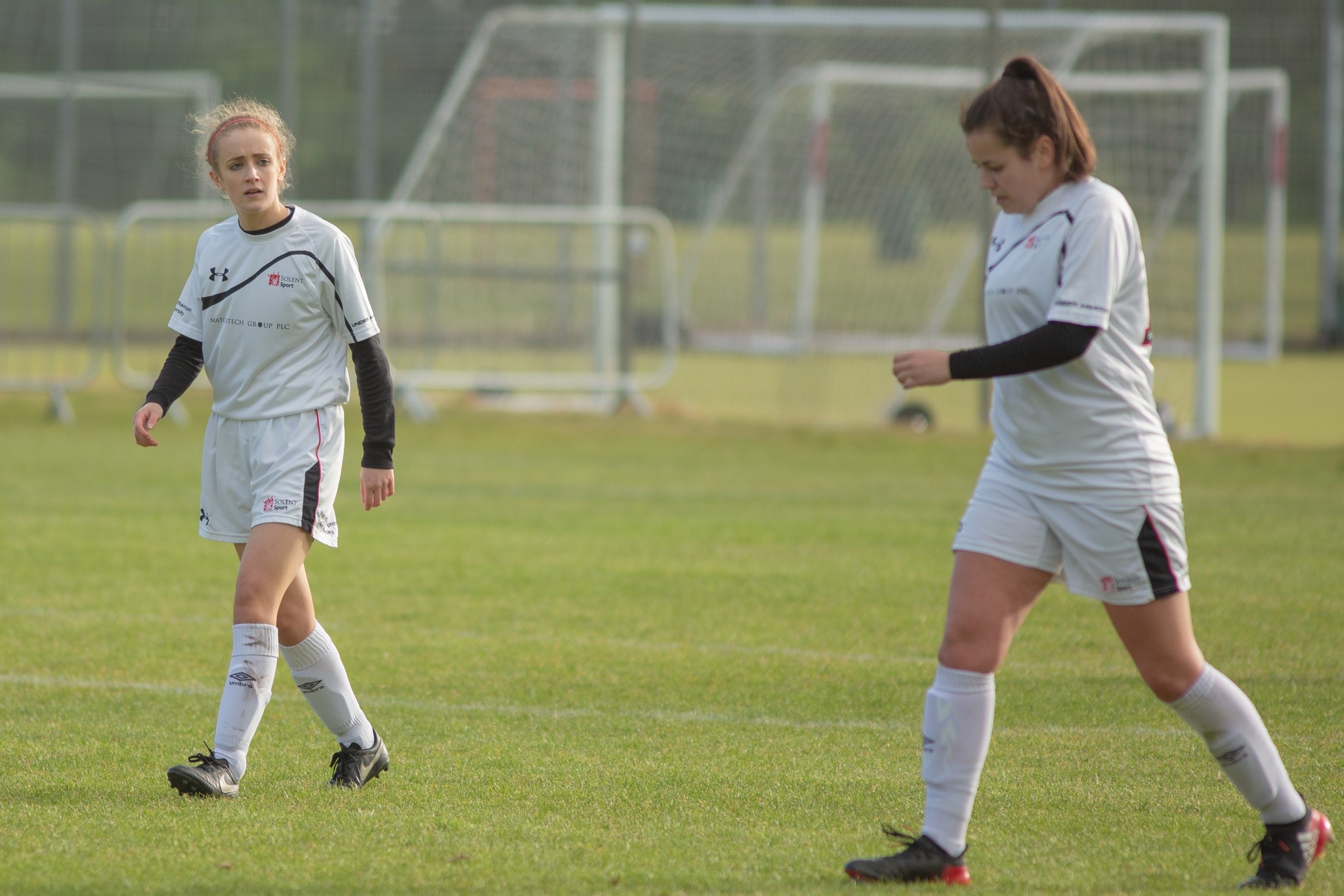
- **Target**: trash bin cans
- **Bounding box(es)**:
[871,185,929,263]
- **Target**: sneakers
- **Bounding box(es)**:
[1239,790,1333,891]
[167,740,239,800]
[326,728,389,788]
[844,821,971,887]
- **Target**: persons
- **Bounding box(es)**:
[843,54,1339,891]
[134,101,396,797]
